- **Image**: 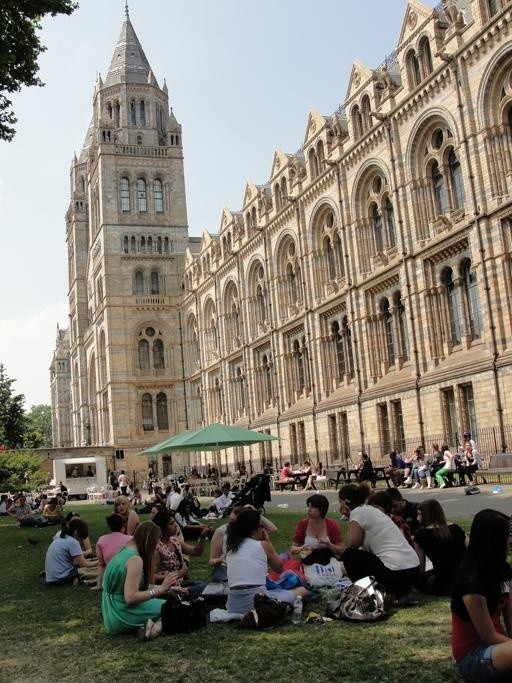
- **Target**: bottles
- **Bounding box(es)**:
[299,541,319,558]
[293,596,302,625]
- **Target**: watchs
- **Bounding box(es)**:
[199,532,208,541]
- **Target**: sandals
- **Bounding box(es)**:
[136,618,154,641]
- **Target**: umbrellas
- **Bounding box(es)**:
[137,424,286,493]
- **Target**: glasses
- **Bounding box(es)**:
[259,523,266,528]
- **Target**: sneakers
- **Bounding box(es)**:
[404,476,412,484]
[420,484,424,489]
[439,483,446,488]
[430,483,435,488]
[426,485,432,489]
[398,484,406,489]
[412,482,419,488]
[468,480,476,486]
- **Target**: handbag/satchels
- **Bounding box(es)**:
[244,593,294,631]
[162,593,209,634]
[328,577,386,621]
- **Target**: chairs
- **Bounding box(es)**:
[187,475,248,498]
[326,465,392,490]
[473,453,512,486]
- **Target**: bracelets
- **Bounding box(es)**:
[149,587,155,598]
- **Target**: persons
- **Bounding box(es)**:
[405,447,430,489]
[458,443,480,486]
[227,509,307,618]
[463,433,475,450]
[91,514,137,591]
[110,497,140,536]
[305,463,326,492]
[222,486,236,509]
[281,462,299,491]
[0,496,9,515]
[206,490,224,519]
[45,519,88,588]
[385,488,423,535]
[131,490,141,504]
[57,494,65,506]
[154,489,167,510]
[209,506,277,586]
[6,496,48,528]
[53,511,92,565]
[263,464,274,479]
[301,462,312,490]
[237,466,248,490]
[168,489,184,515]
[174,501,214,540]
[358,456,376,487]
[42,498,61,525]
[451,509,512,683]
[102,522,178,642]
[147,513,213,603]
[368,491,413,550]
[147,468,154,490]
[420,443,444,489]
[87,466,94,477]
[72,467,79,480]
[435,446,456,488]
[182,486,194,503]
[290,496,343,562]
[108,472,117,490]
[413,499,471,595]
[60,482,68,498]
[191,469,201,483]
[386,453,407,487]
[338,485,419,605]
[118,470,127,496]
